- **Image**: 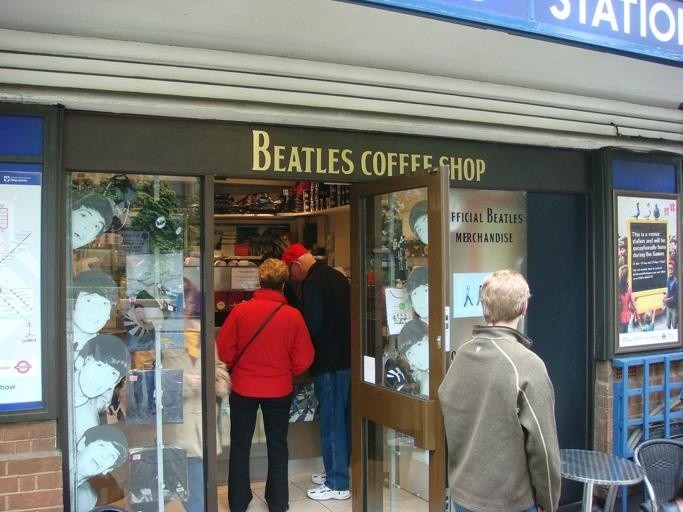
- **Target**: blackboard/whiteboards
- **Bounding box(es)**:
[626,217,669,297]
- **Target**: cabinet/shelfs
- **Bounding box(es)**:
[212,203,350,261]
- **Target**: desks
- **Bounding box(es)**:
[560,448,648,512]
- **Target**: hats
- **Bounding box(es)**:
[283,242,308,265]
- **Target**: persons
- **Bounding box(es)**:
[438,270,562,512]
[217,258,315,512]
[160,288,232,511]
[281,244,352,501]
[618,233,678,335]
[397,199,429,398]
[667,461,683,512]
[69,186,156,512]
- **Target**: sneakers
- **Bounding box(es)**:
[311,470,328,485]
[267,502,290,512]
[306,486,352,501]
[229,492,253,512]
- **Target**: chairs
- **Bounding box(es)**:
[633,438,683,511]
